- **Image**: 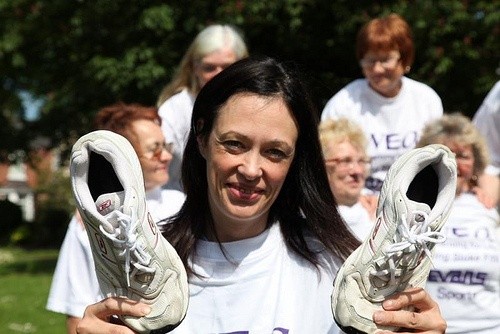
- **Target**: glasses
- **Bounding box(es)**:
[452,150,475,166]
[360,57,400,69]
[127,142,173,161]
[324,157,371,170]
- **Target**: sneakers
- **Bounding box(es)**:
[331,143,457,334]
[70,130,189,334]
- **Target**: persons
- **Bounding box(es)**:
[413,114,500,333]
[46,104,187,334]
[318,118,378,245]
[158,25,251,193]
[76,53,447,334]
[322,17,443,199]
